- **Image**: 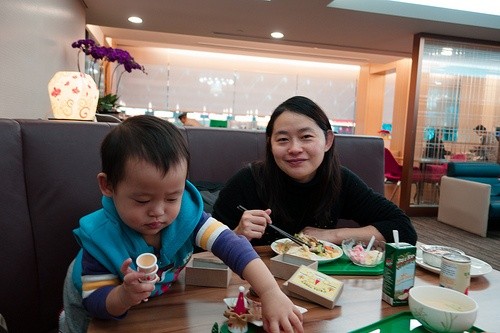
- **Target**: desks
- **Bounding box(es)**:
[395,156,448,203]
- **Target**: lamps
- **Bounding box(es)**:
[48,71,99,119]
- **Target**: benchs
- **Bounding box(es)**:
[0,119,391,333]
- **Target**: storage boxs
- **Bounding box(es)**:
[270,254,343,309]
[185,258,231,287]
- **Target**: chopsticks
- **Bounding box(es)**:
[238,205,311,248]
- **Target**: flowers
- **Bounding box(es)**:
[72,39,148,111]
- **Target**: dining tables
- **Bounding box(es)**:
[87,251,500,333]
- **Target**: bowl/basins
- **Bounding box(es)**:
[342,237,386,267]
[419,245,465,269]
[408,286,478,333]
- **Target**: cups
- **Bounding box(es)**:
[136,253,159,284]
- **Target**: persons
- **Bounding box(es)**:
[178,112,187,124]
[58,116,304,333]
[470,125,500,162]
[415,129,451,196]
[212,96,417,246]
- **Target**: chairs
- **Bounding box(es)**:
[384,148,465,205]
[437,162,500,237]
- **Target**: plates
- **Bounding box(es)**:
[223,298,307,327]
[271,238,343,264]
[415,250,492,277]
